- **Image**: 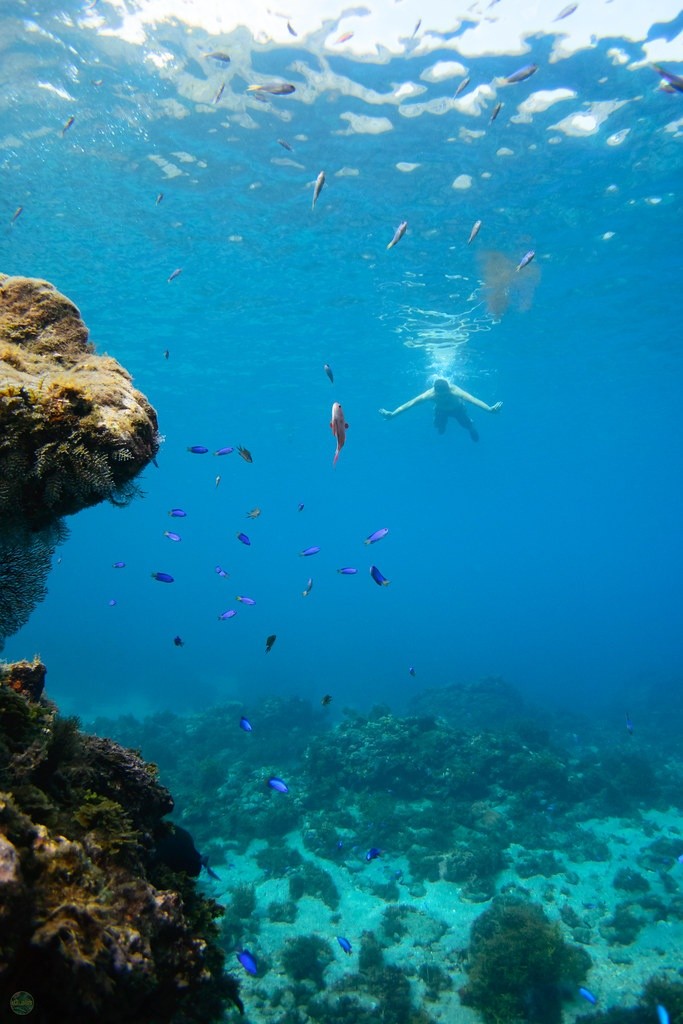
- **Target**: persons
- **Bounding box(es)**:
[379,379,503,441]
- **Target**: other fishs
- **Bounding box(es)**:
[0,0,683,1024]
